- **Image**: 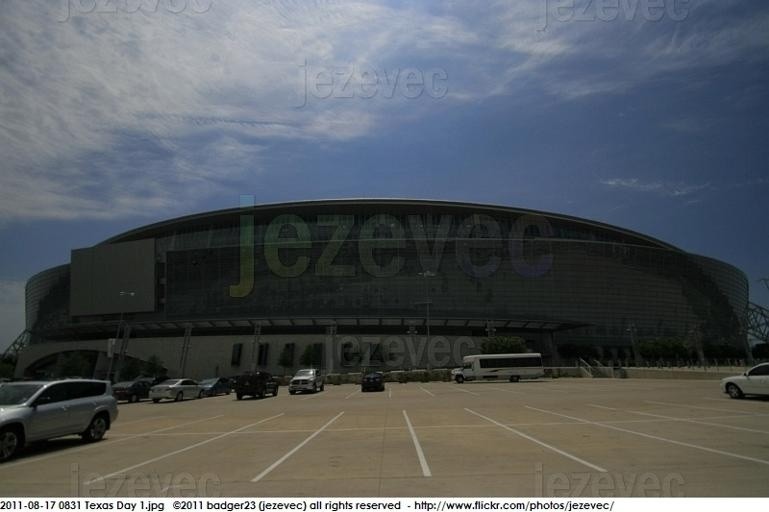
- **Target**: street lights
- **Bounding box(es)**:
[109,290,135,375]
[416,270,436,366]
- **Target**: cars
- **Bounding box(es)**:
[361,371,384,392]
[109,380,152,402]
[148,376,231,403]
[720,363,769,399]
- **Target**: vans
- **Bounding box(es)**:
[451,352,544,384]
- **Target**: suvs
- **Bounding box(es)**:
[288,369,324,395]
[235,372,279,400]
[0,378,118,460]
